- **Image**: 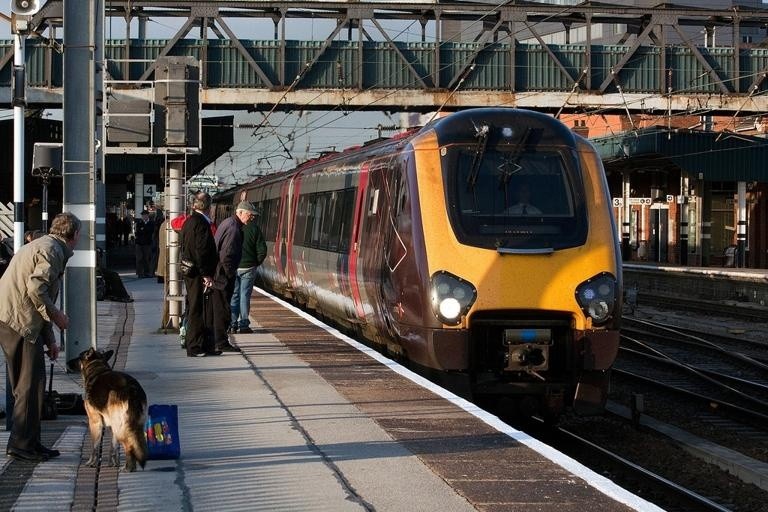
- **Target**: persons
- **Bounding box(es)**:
[1,212,82,461]
[104,192,267,357]
[24,230,48,244]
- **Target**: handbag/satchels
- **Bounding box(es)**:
[144,404,180,460]
[179,258,193,276]
[40,390,84,420]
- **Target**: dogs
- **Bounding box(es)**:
[65,346,149,474]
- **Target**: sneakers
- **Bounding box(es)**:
[231,329,253,333]
[187,345,240,357]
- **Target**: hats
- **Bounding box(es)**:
[237,201,259,216]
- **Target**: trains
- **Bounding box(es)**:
[209,106,628,439]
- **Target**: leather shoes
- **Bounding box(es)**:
[7,444,60,462]
